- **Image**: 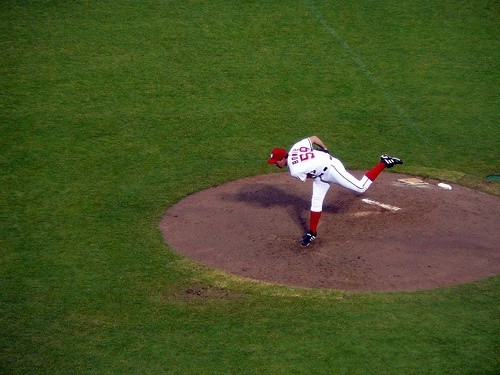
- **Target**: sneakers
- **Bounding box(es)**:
[299,232,317,247]
[381,155,403,169]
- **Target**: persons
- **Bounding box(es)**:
[266,135,404,247]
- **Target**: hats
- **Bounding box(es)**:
[267,148,287,164]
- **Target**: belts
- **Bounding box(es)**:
[318,156,332,177]
